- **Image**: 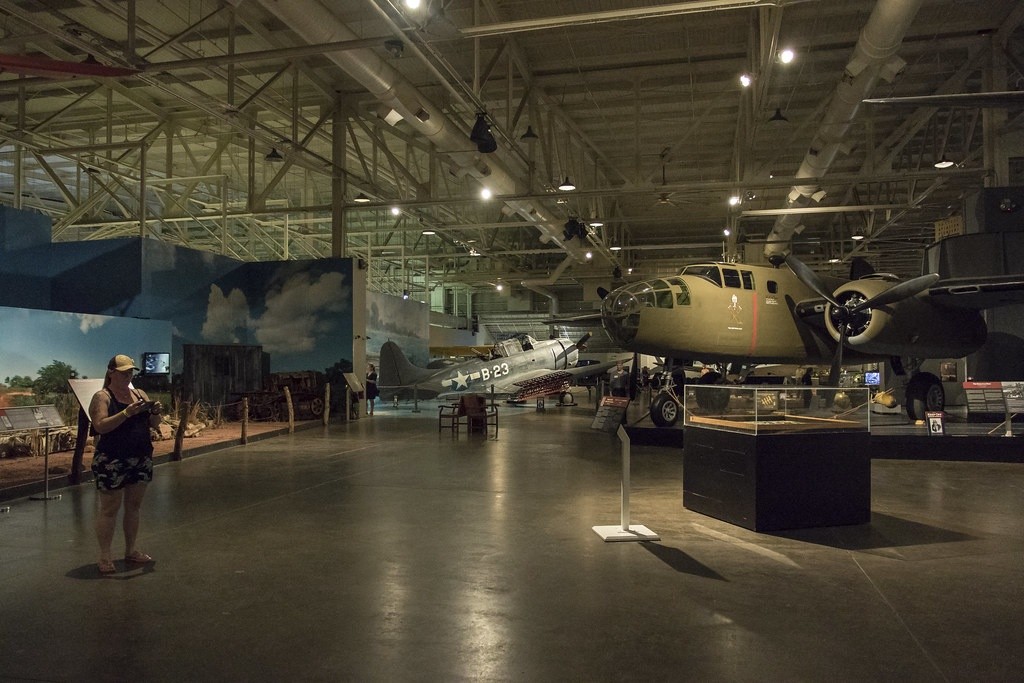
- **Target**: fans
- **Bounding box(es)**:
[647,147,692,211]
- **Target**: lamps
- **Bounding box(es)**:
[767,93,789,127]
[78,26,103,66]
[851,231,863,240]
[264,147,283,162]
[1000,197,1016,212]
[590,155,604,228]
[558,131,576,190]
[415,108,430,124]
[520,81,538,143]
[934,154,954,168]
[354,193,370,203]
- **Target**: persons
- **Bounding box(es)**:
[609,360,631,425]
[801,367,814,408]
[88,354,163,573]
[366,362,377,416]
[701,365,709,377]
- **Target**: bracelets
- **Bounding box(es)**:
[122,409,132,418]
[150,409,161,416]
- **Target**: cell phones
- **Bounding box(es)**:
[143,401,155,409]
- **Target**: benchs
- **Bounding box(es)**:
[464,398,502,440]
[438,396,465,434]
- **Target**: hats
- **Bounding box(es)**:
[108,354,140,372]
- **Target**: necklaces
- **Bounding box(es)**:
[114,389,130,398]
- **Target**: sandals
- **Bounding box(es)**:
[98,558,115,572]
[126,551,152,562]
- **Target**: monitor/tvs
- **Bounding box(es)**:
[144,352,170,375]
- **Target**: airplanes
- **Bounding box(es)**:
[541,250,1024,428]
[377,332,635,405]
